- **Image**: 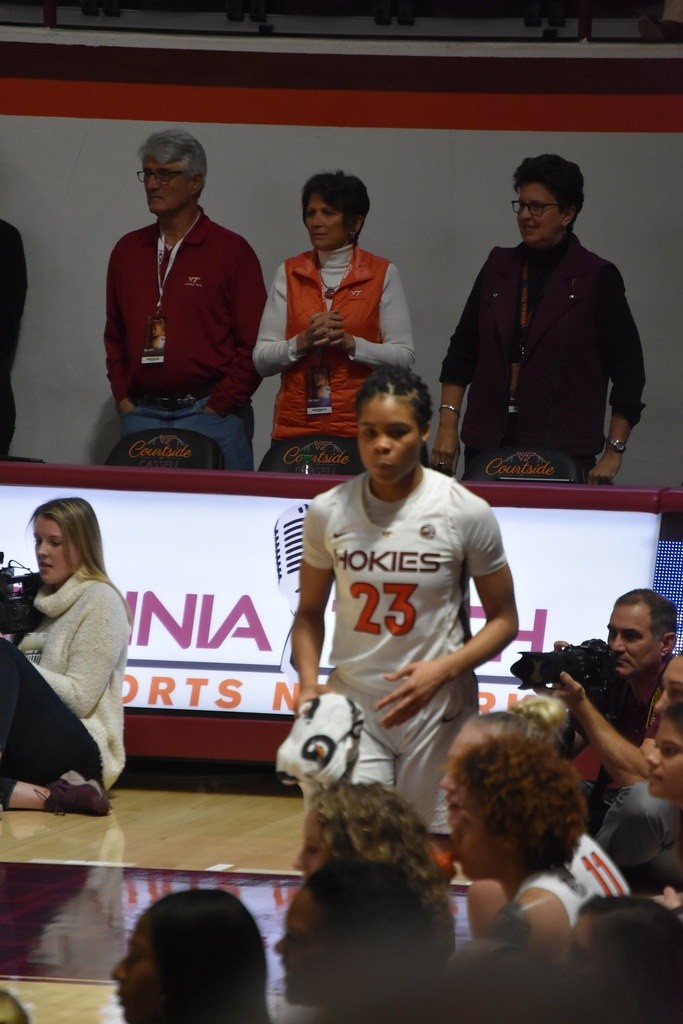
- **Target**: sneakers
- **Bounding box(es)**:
[34,771,110,816]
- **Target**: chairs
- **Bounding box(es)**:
[259,431,367,475]
[102,426,226,471]
[460,444,588,484]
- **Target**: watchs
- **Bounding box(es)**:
[607,437,627,453]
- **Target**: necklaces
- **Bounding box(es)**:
[320,263,340,300]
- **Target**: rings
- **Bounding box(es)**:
[439,462,445,466]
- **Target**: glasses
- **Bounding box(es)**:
[511,200,560,216]
[137,170,190,183]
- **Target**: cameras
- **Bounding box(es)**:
[511,638,621,700]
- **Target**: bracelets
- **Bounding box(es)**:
[439,404,460,417]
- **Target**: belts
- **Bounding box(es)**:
[130,391,210,411]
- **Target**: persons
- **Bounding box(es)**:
[252,170,416,475]
[0,497,132,817]
[105,128,267,471]
[0,220,28,459]
[432,155,646,485]
[279,363,519,882]
[110,589,683,1024]
